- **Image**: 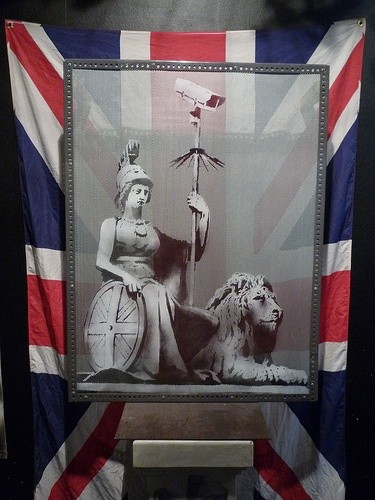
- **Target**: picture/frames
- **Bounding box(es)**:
[60,58,328,405]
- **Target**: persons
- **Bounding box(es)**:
[94,163,220,381]
